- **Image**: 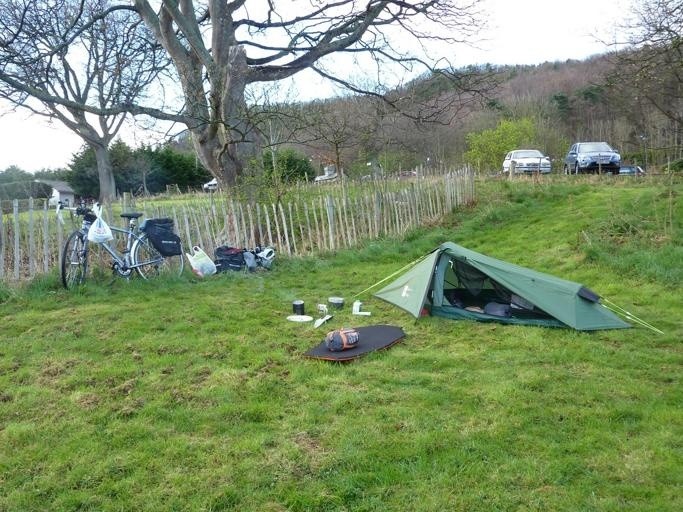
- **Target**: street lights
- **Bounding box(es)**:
[213,245,244,271]
[150,231,182,257]
[138,216,175,238]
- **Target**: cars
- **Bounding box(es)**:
[292,300,305,316]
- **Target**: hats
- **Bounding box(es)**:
[390,171,422,179]
[502,150,551,175]
[201,178,217,193]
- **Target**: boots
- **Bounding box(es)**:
[607,165,648,176]
[563,142,620,176]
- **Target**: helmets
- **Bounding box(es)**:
[285,315,313,322]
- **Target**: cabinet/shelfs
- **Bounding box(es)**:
[352,299,362,313]
